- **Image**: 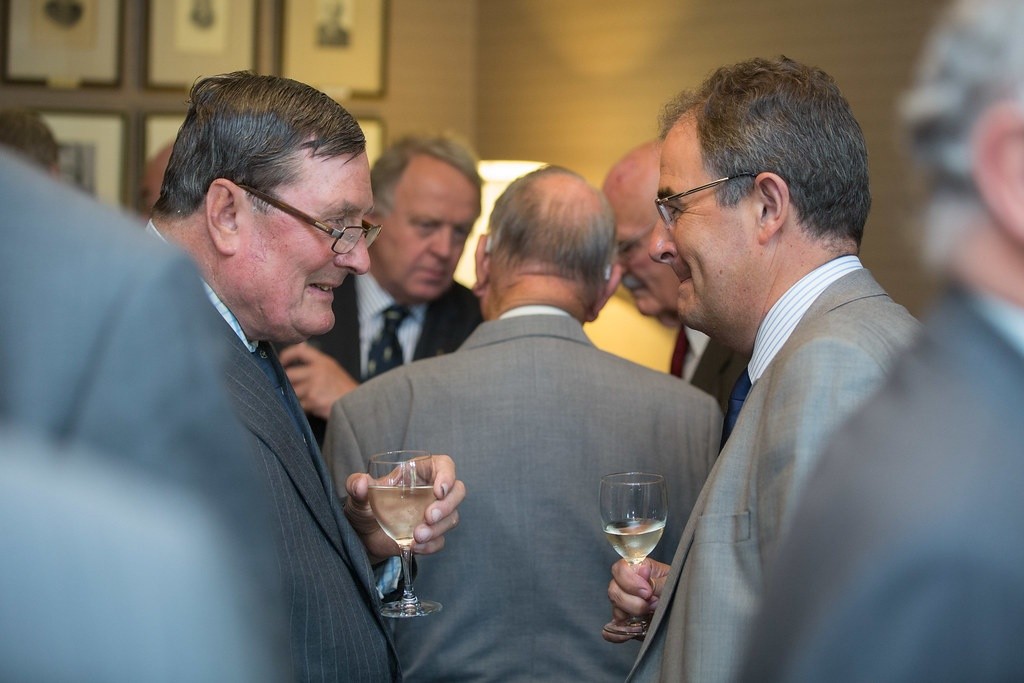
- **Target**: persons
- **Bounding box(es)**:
[0,0,1023,683]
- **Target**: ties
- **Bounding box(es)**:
[368,304,408,378]
[718,367,752,457]
[671,323,689,378]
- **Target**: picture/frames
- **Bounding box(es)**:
[0,0,391,214]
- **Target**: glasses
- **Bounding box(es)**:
[238,184,383,254]
[616,221,656,266]
[655,172,790,231]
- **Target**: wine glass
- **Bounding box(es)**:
[364,450,443,618]
[599,472,668,635]
